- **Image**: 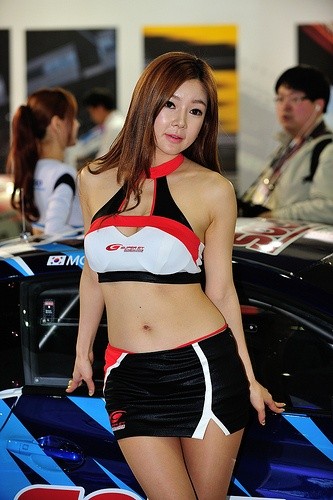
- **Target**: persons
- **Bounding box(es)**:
[239,65,332,225]
[5,88,84,237]
[84,87,127,161]
[66,51,286,500]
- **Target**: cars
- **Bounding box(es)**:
[0,214,332,500]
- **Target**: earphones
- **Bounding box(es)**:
[315,104,320,112]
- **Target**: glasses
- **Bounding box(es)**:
[272,93,309,105]
